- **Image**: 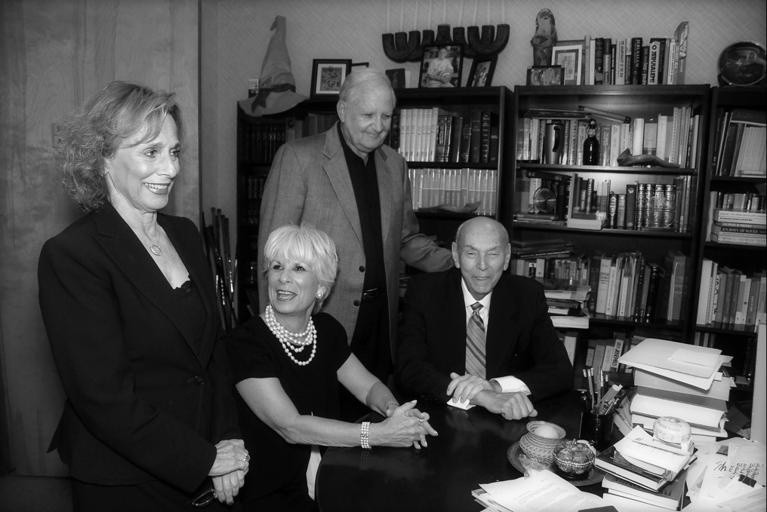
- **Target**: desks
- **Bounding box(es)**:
[314,388,752,512]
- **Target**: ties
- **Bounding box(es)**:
[465,303,487,378]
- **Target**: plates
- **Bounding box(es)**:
[505,440,604,487]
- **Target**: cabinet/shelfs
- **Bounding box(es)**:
[689,83,767,370]
[508,86,711,370]
[383,85,507,222]
[236,97,341,313]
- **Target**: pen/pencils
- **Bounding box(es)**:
[576,367,628,417]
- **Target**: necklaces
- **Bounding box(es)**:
[265,305,317,365]
[116,207,162,256]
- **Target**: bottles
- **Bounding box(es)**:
[582,119,599,165]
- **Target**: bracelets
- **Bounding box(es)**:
[361,422,373,448]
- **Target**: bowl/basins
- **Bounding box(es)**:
[553,452,595,476]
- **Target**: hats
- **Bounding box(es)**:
[238,16,309,117]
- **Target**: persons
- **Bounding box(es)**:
[38,82,250,512]
[257,69,451,388]
[422,47,488,87]
[237,226,438,512]
[397,218,574,420]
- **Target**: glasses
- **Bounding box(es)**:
[192,489,216,506]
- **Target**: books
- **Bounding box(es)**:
[694,112,767,377]
[387,107,500,218]
[238,114,337,314]
[583,36,686,84]
[513,106,699,373]
[472,338,736,512]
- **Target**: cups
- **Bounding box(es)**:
[580,409,614,451]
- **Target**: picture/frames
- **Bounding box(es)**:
[465,53,498,88]
[551,41,583,85]
[418,43,463,88]
[310,59,351,96]
